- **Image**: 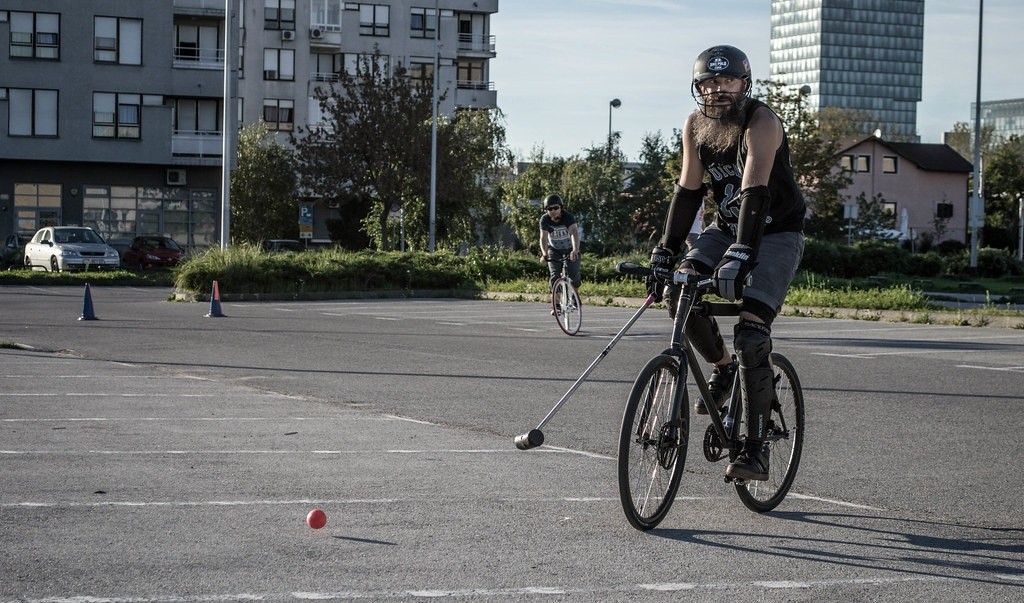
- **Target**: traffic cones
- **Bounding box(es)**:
[77,283,100,321]
[202,281,228,318]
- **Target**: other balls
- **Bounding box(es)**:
[306,509,327,529]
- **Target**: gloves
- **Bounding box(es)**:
[712,243,757,301]
[647,246,680,303]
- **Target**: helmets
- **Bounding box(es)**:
[544,194,564,209]
[691,46,752,120]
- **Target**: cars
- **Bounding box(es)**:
[122,235,186,270]
[23,226,121,273]
[3,230,36,266]
[264,239,333,253]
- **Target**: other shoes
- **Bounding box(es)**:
[552,306,562,317]
[572,293,582,308]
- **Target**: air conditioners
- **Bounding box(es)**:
[312,29,326,39]
[282,31,295,41]
[167,169,186,186]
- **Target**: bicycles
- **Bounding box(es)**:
[615,258,805,534]
[545,254,583,336]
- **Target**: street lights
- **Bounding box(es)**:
[609,98,621,165]
[798,84,812,140]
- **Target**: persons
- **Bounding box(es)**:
[644,46,806,479]
[539,194,583,315]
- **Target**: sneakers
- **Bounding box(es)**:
[695,355,739,414]
[726,440,771,481]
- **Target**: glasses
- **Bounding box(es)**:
[548,206,559,211]
